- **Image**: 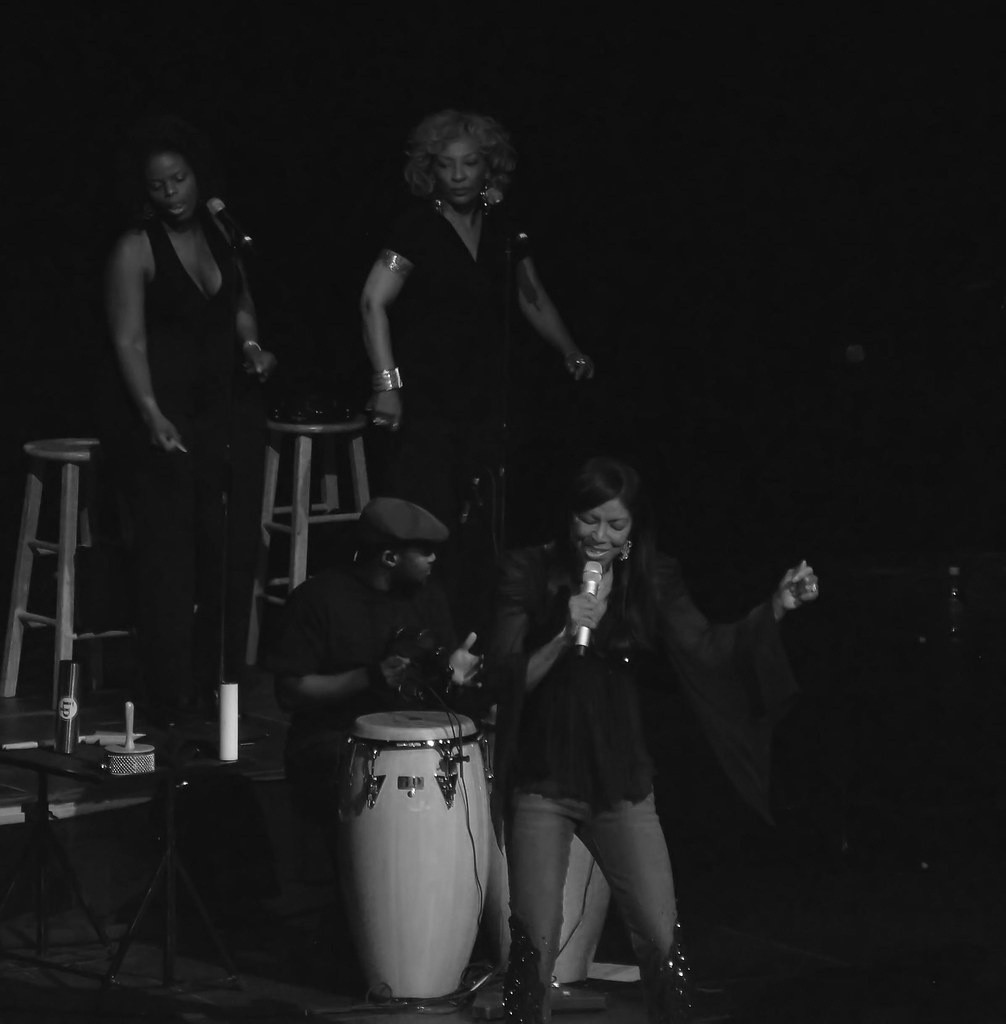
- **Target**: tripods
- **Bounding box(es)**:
[95,744,241,999]
[0,753,112,959]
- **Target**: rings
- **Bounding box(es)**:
[812,584,817,592]
[577,360,585,365]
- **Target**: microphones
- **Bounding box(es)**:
[207,197,254,249]
[574,560,603,658]
[484,188,529,248]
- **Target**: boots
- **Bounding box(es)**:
[500,915,564,1024]
[628,922,696,1024]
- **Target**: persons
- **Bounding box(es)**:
[273,497,478,990]
[84,112,276,736]
[357,111,598,551]
[488,454,821,1024]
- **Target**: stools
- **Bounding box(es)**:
[4,438,101,707]
[244,408,372,671]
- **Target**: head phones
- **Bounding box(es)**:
[389,622,454,707]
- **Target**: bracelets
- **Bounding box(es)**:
[242,340,262,354]
[370,368,403,392]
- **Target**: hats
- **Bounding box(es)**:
[358,497,446,547]
